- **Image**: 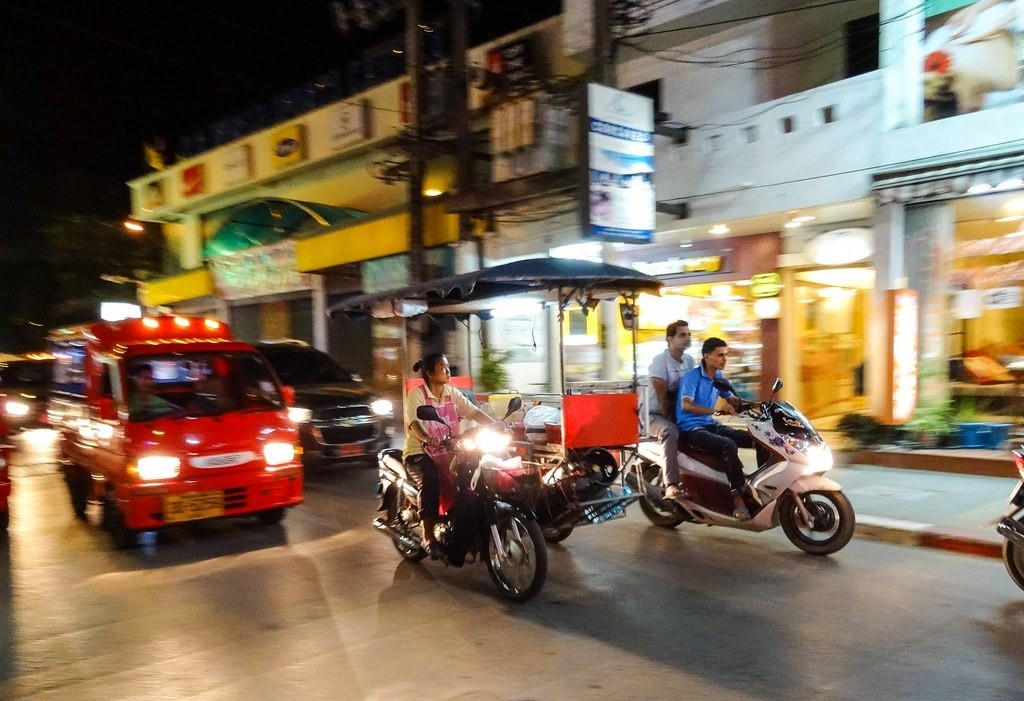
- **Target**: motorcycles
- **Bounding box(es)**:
[626,378,856,555]
[327,257,664,603]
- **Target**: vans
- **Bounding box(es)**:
[44,315,304,547]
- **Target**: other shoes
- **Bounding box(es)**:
[665,487,690,500]
[733,508,750,523]
[421,539,444,559]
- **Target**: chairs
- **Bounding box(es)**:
[187,380,233,412]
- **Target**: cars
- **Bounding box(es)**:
[0,353,52,426]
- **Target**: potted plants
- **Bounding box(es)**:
[901,398,958,447]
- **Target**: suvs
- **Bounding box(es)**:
[243,339,391,481]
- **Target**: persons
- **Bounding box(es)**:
[674,337,772,520]
[126,365,171,417]
[401,352,509,559]
[638,319,696,500]
